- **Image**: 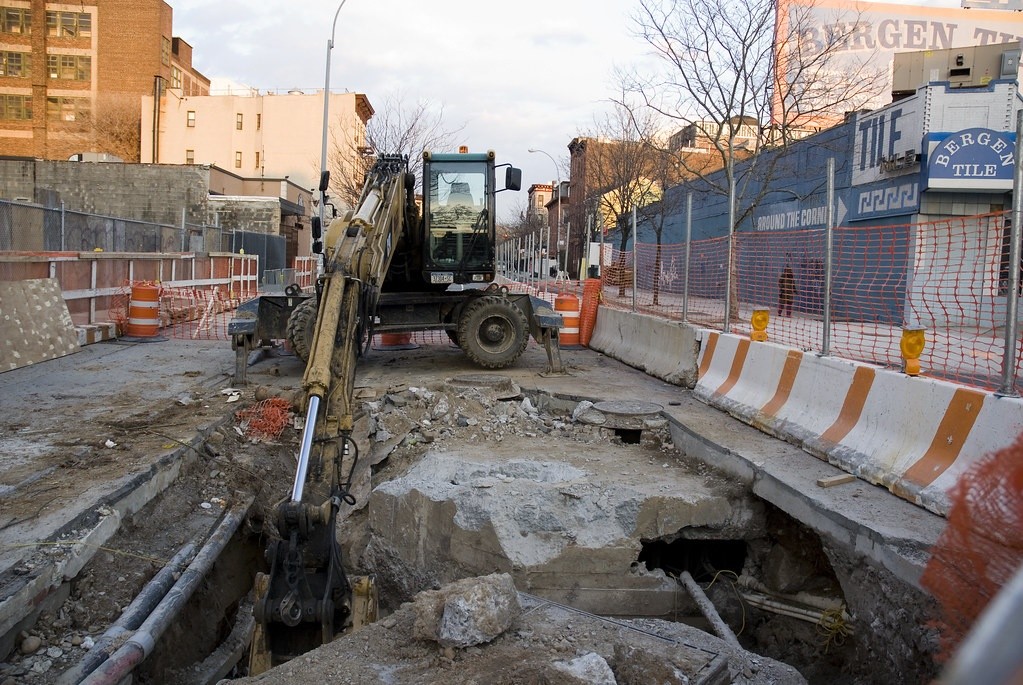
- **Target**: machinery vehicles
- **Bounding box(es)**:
[226,142,577,676]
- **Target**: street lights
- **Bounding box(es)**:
[528,148,560,271]
